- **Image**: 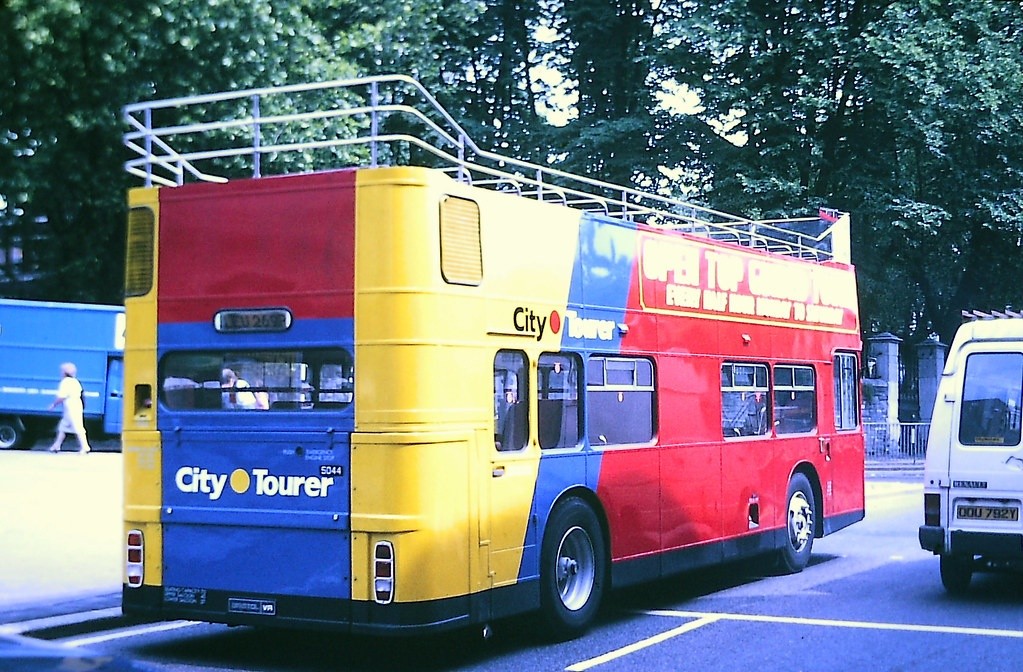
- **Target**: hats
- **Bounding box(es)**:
[61,362,78,378]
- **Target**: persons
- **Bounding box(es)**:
[47,361,91,455]
[218,368,270,411]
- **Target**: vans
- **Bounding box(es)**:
[920,306,1022,596]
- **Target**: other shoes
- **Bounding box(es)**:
[47,446,61,452]
[79,447,91,453]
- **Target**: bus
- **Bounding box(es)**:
[123,75,869,642]
